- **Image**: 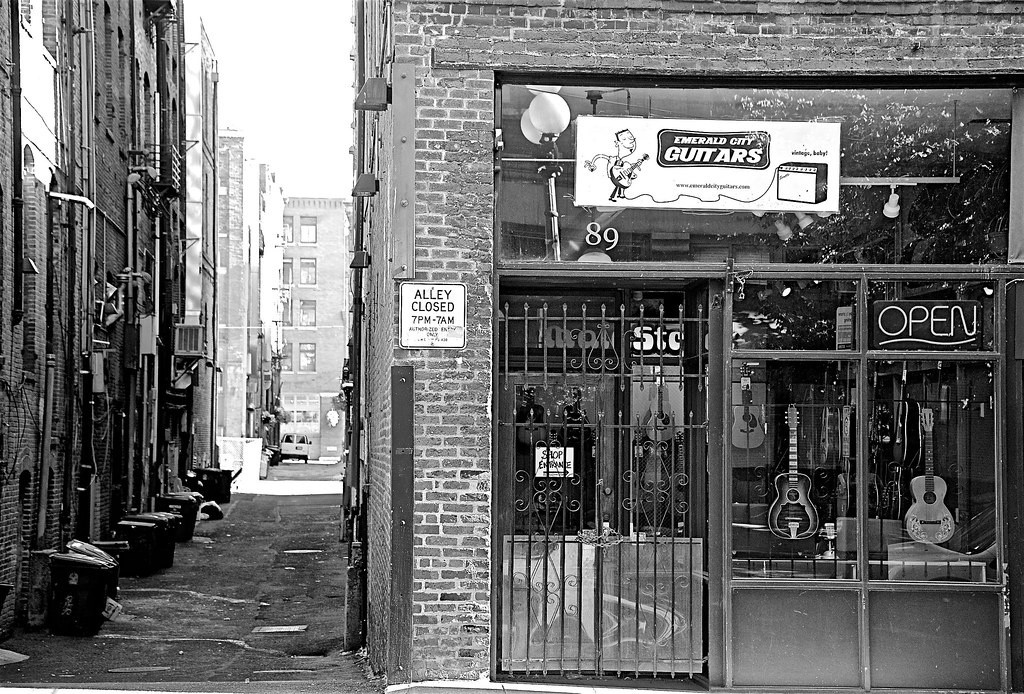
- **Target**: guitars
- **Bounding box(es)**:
[904,408,955,545]
[645,388,676,441]
[561,386,592,442]
[767,403,819,541]
[549,428,559,447]
[893,360,923,468]
[732,362,765,450]
[516,386,548,446]
[820,359,842,465]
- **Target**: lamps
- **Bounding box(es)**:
[774,214,793,240]
[354,77,392,111]
[351,173,380,197]
[794,212,814,230]
[350,251,371,268]
[578,90,612,263]
[884,187,901,218]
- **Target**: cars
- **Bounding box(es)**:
[280,432,312,464]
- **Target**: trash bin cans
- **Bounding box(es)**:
[193,466,234,504]
[157,491,205,544]
[65,534,130,603]
[48,552,107,638]
[260,445,281,479]
[117,510,184,577]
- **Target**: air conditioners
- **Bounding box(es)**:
[174,323,205,358]
